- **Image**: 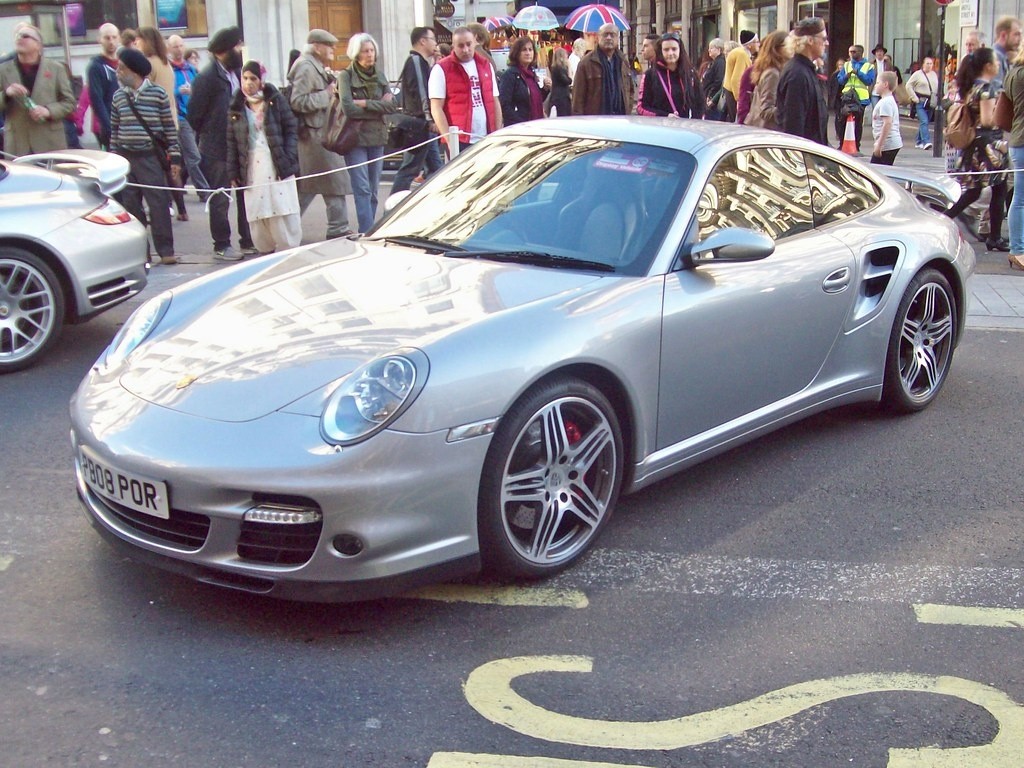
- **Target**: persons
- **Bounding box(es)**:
[0,18,1024,279]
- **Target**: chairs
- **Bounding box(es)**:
[552,153,645,259]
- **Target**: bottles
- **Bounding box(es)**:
[21,93,45,125]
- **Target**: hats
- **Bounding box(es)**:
[208,24,241,54]
[242,60,260,79]
[872,44,887,56]
[740,29,757,45]
[794,17,826,37]
[116,46,152,76]
[307,29,339,44]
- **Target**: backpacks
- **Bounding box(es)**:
[840,72,863,117]
[943,84,980,151]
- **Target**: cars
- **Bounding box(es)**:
[69,115,976,603]
[0,148,148,374]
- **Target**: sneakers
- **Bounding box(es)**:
[212,246,244,261]
[239,246,257,254]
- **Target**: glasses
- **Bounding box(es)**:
[849,51,859,54]
[15,33,39,44]
[322,42,336,47]
[661,34,681,43]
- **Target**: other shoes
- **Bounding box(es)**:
[177,213,189,221]
[917,143,1024,272]
[161,254,179,266]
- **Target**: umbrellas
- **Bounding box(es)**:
[563,0,631,51]
[482,15,512,36]
[511,2,559,45]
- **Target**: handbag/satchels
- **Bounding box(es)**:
[77,106,100,150]
[276,58,331,141]
[923,98,934,112]
[322,69,364,156]
[382,112,430,155]
[991,89,1015,133]
[151,130,172,172]
